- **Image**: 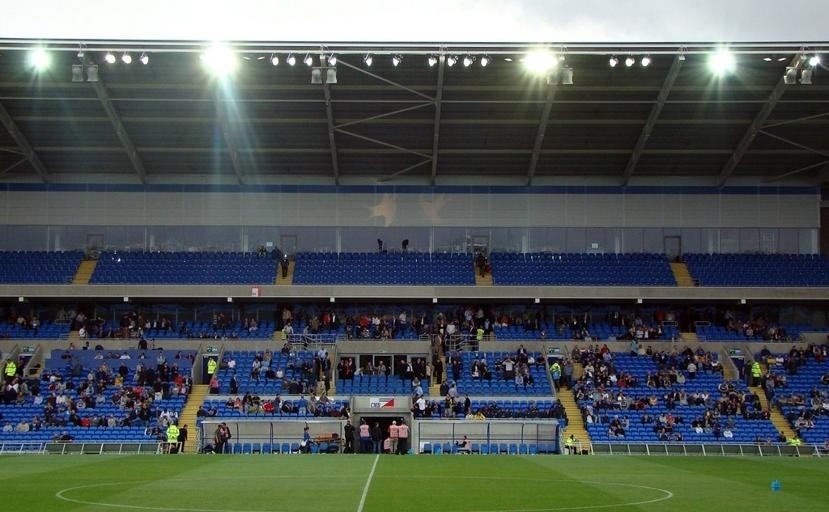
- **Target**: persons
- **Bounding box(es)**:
[280,253,290,278]
[401,239,410,257]
[271,245,284,260]
[475,252,488,277]
[377,237,383,253]
[1,303,827,456]
[257,244,268,259]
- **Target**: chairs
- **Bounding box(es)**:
[0,300,827,341]
[0,345,827,455]
[0,252,828,289]
